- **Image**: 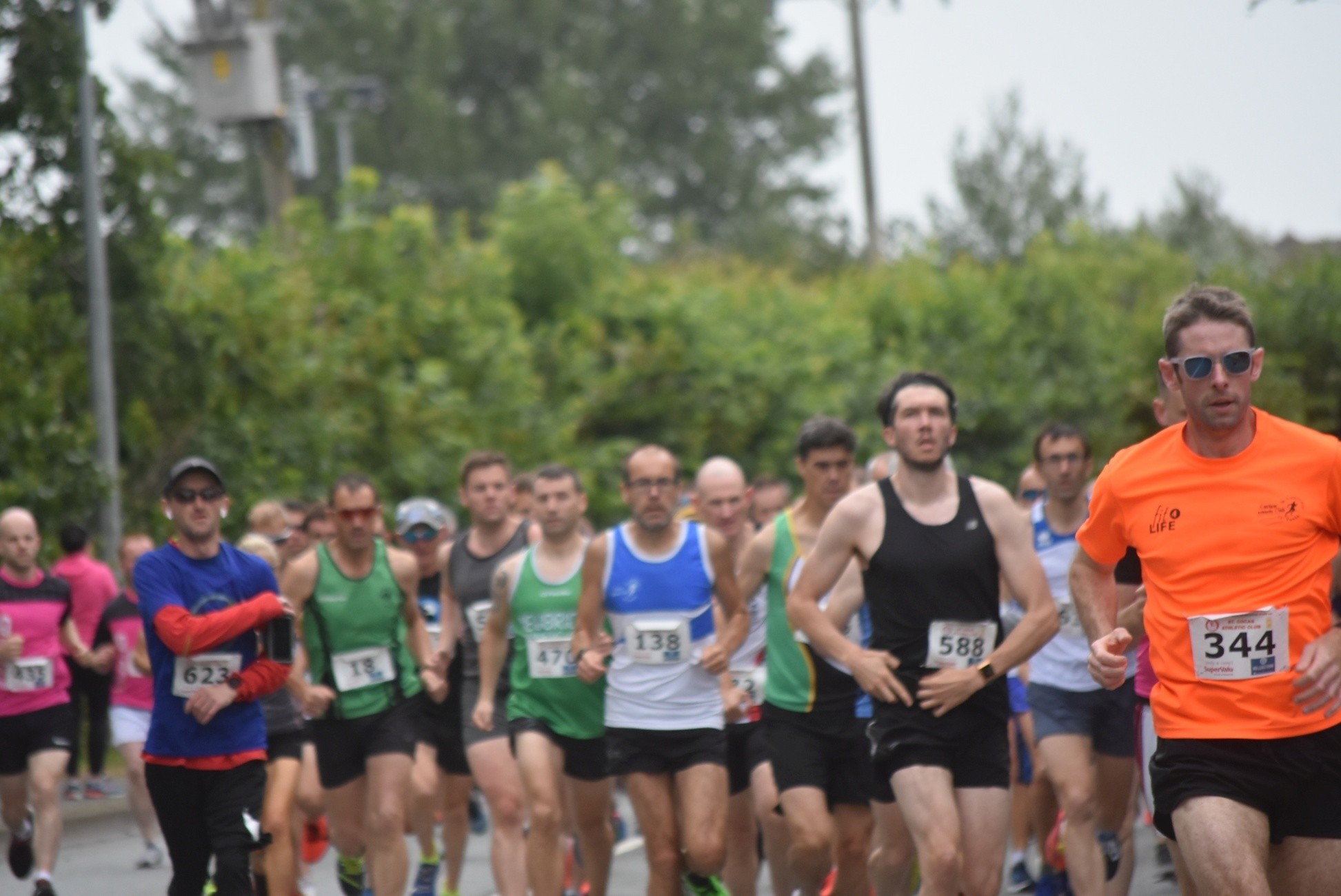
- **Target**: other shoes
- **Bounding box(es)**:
[135,843,166,868]
[682,873,730,895]
[33,873,56,896]
[299,813,330,863]
[409,845,443,896]
[84,777,127,800]
[64,778,84,803]
[5,806,37,879]
[1095,835,1121,880]
[466,799,488,836]
[1148,843,1176,883]
[336,849,365,896]
[1006,864,1034,894]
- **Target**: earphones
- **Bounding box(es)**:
[220,508,226,518]
[166,509,172,520]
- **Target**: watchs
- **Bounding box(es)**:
[976,659,997,684]
[224,676,250,697]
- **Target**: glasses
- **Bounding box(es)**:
[1171,348,1254,381]
[334,506,375,523]
[172,483,225,505]
[272,533,294,547]
[403,527,438,543]
[1016,489,1045,502]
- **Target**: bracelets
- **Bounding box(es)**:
[574,645,598,661]
[438,652,453,662]
[1335,622,1341,629]
[420,664,440,671]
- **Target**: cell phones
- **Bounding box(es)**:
[267,615,293,664]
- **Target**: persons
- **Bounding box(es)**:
[0,506,93,896]
[1070,284,1341,896]
[50,355,1341,896]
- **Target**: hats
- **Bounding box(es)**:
[392,496,448,535]
[163,454,224,490]
